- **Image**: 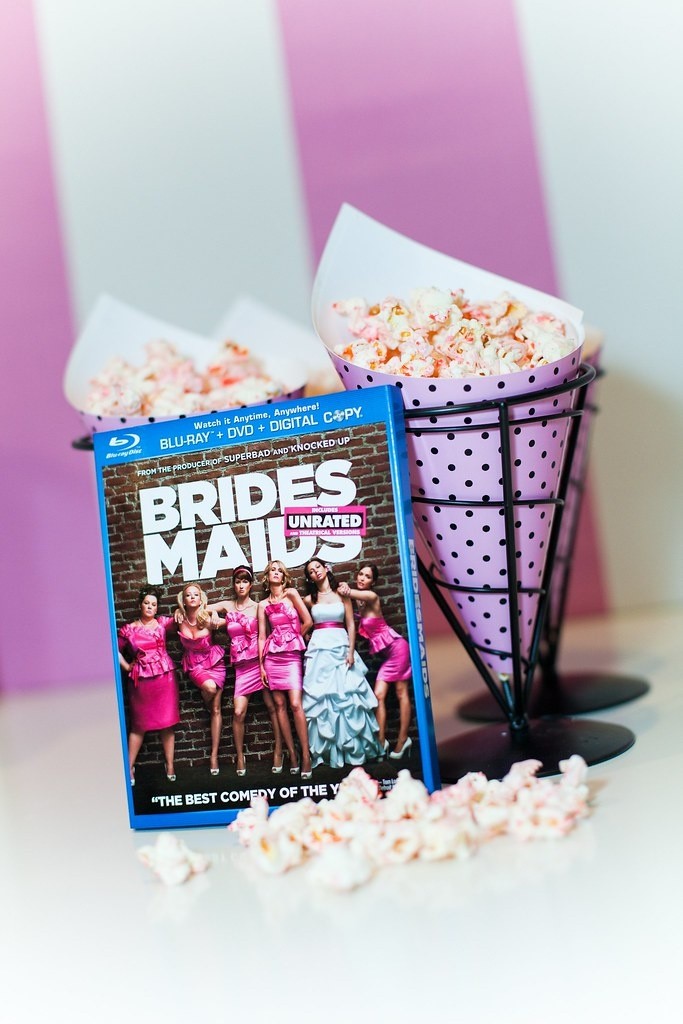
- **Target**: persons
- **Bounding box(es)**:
[337,564,412,759]
[258,560,313,779]
[302,557,385,769]
[118,586,184,786]
[174,583,226,775]
[206,565,284,776]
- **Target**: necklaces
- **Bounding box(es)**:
[235,598,251,611]
[139,619,144,626]
[270,591,283,604]
[318,589,332,595]
[186,617,196,626]
[359,601,365,610]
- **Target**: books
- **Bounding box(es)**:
[92,385,440,829]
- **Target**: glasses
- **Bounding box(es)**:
[142,590,156,595]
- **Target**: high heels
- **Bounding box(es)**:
[164,763,176,781]
[210,768,220,776]
[289,753,301,775]
[236,756,246,776]
[390,737,412,760]
[301,771,312,780]
[272,754,284,774]
[130,766,136,787]
[376,739,390,758]
